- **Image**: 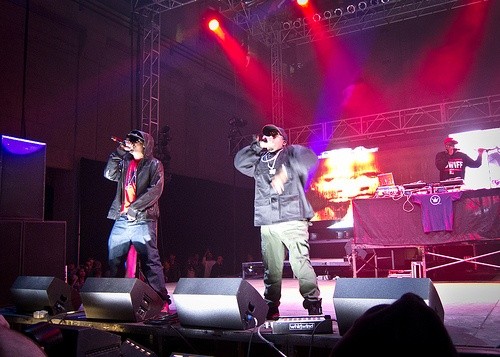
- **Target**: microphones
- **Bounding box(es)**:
[453,148,460,150]
[257,137,267,142]
[111,136,126,147]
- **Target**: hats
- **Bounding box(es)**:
[131,128,152,148]
[443,136,458,143]
[261,122,290,142]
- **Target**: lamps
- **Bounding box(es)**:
[228,116,247,127]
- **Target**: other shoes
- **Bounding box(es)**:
[157,293,170,316]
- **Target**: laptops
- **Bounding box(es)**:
[378,172,395,186]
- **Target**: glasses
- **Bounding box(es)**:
[263,129,280,137]
[129,136,141,143]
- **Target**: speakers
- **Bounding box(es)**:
[0,135,46,221]
[80,277,164,323]
[0,221,22,307]
[173,277,269,330]
[9,276,81,317]
[50,323,122,357]
[22,221,67,285]
[333,278,444,336]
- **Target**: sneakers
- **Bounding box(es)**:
[266,296,281,320]
[301,298,323,314]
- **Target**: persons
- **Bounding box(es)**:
[433,136,484,184]
[103,129,172,313]
[71,253,254,288]
[234,124,322,320]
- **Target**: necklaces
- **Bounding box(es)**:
[260,149,282,174]
[128,170,136,183]
